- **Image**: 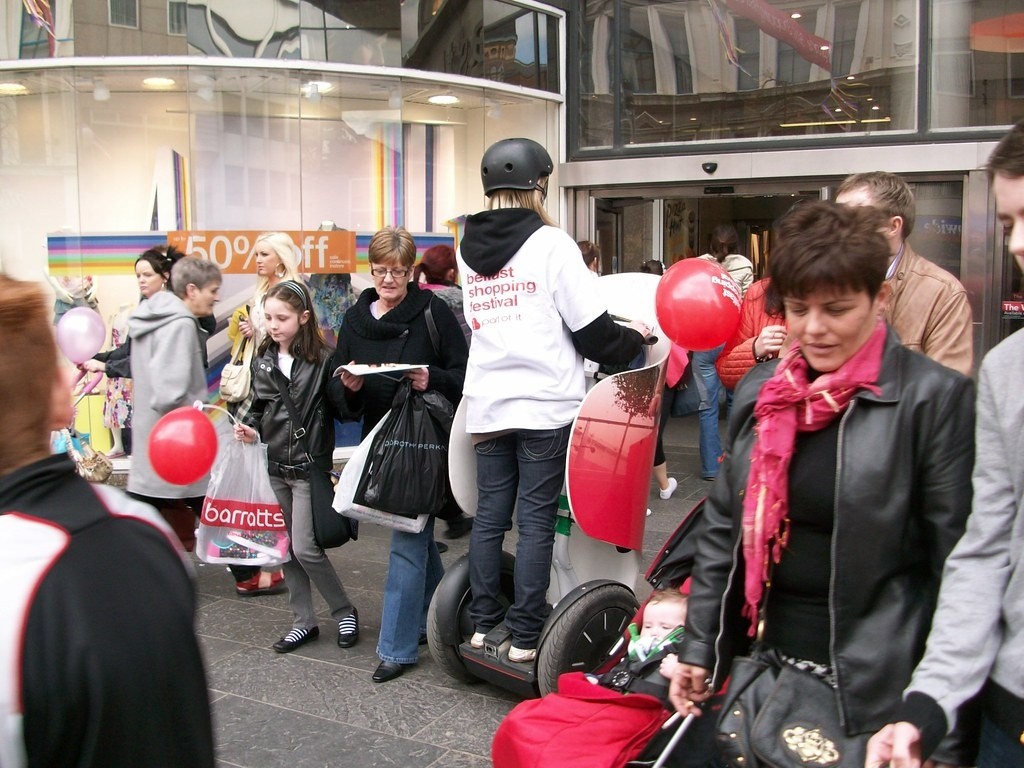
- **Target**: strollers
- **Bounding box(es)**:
[491,452,727,768]
[51,367,104,457]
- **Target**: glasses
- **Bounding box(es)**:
[370,262,410,279]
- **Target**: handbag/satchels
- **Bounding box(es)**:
[62,427,113,482]
[353,378,453,521]
[331,408,429,533]
[309,453,352,548]
[195,433,290,568]
[712,654,887,767]
[219,332,254,402]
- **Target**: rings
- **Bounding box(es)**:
[773,333,775,338]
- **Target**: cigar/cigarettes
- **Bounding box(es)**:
[662,702,694,729]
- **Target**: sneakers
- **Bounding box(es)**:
[206,539,269,565]
[227,522,288,557]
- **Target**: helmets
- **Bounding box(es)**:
[480,137,553,197]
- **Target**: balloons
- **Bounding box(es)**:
[655,258,743,350]
[57,306,106,365]
[149,407,218,484]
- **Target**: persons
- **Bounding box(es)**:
[697,224,754,481]
[45,266,102,322]
[227,234,300,424]
[492,593,688,768]
[669,120,1024,768]
[81,247,183,379]
[578,240,599,274]
[326,232,470,683]
[0,275,220,767]
[413,246,472,349]
[639,261,676,499]
[233,280,359,651]
[101,302,133,458]
[127,258,285,597]
[455,137,649,662]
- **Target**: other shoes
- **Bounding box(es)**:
[660,477,677,499]
[471,632,488,648]
[508,646,537,662]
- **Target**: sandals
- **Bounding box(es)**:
[236,570,289,596]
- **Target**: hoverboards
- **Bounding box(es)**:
[426,272,672,698]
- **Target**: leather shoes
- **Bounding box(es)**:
[273,625,320,653]
[338,607,360,647]
[373,661,406,681]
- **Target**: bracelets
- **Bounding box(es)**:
[756,354,772,361]
